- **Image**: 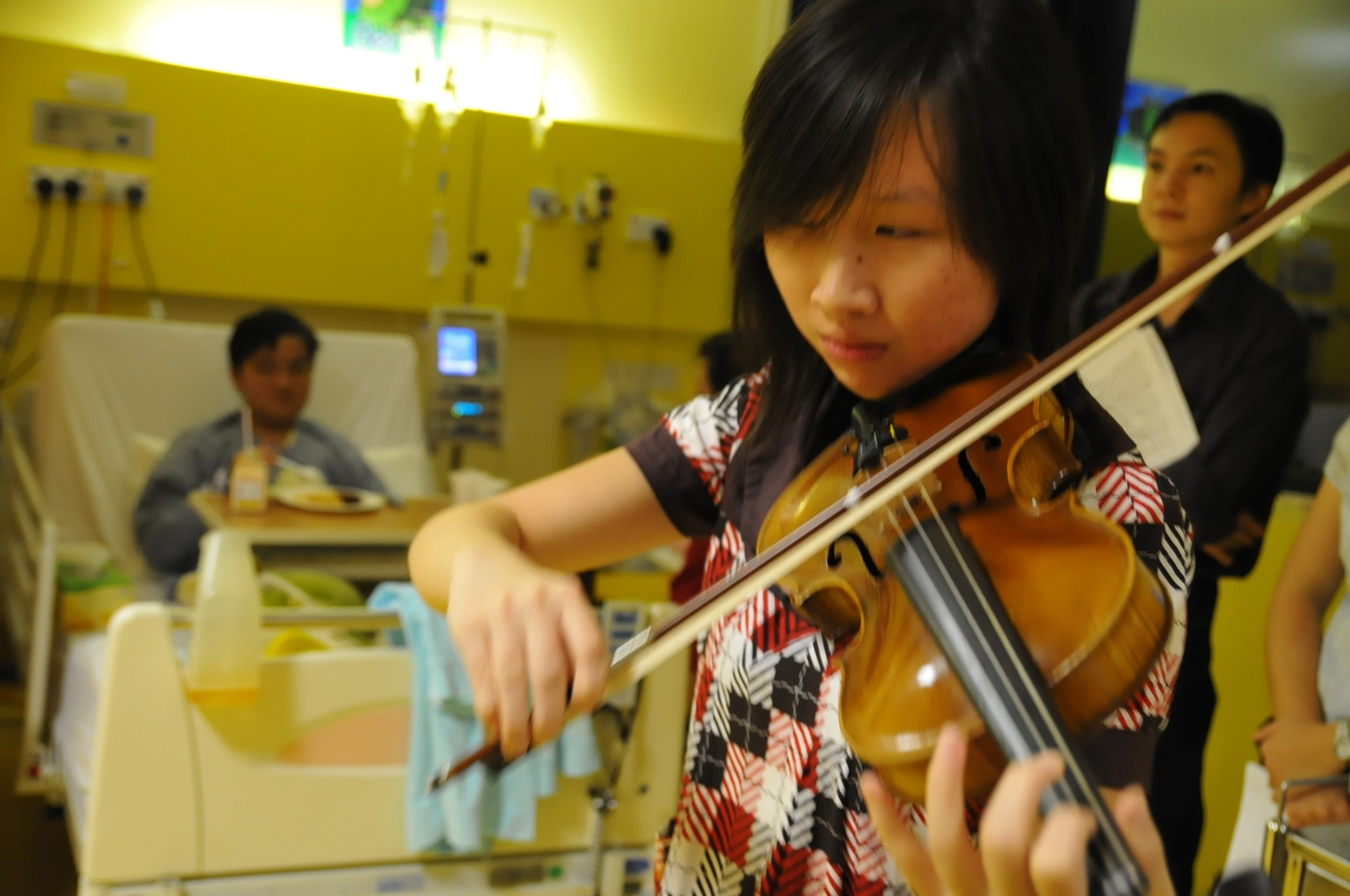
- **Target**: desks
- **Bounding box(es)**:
[189,487,453,579]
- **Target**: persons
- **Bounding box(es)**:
[1049,93,1310,896]
[402,0,1195,895]
[691,328,741,400]
[132,290,392,615]
[1253,415,1350,871]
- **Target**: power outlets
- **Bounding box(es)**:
[25,169,86,200]
[104,173,150,207]
[626,212,667,244]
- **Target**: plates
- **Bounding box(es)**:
[274,484,388,514]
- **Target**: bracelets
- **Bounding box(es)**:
[1333,717,1350,763]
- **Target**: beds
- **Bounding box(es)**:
[2,312,692,895]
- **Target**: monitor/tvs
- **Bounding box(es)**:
[425,302,507,388]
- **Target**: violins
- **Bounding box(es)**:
[751,346,1174,896]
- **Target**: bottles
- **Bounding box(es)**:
[228,446,269,515]
